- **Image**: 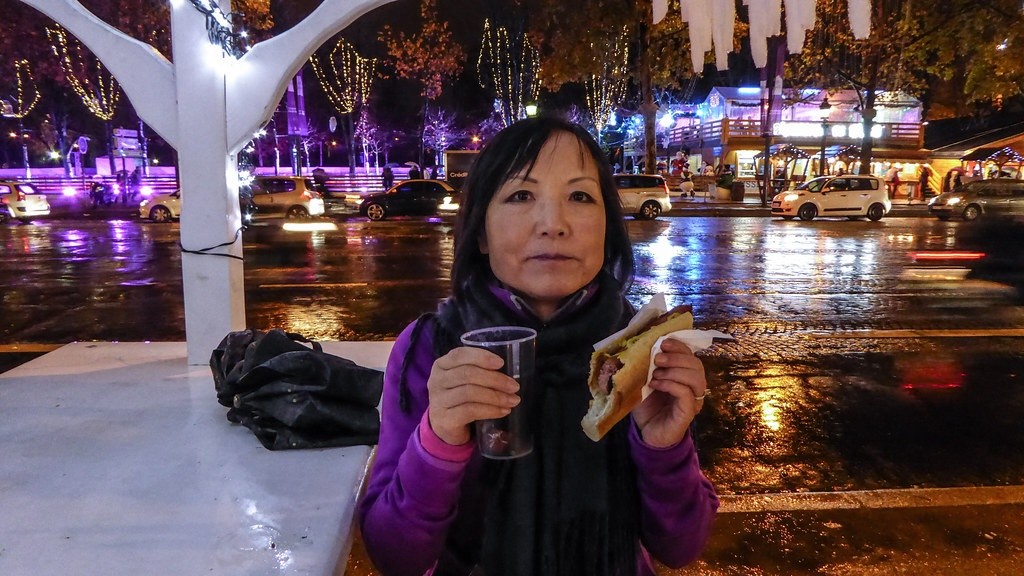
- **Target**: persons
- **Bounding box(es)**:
[89,165,141,207]
[944,171,951,192]
[774,169,785,193]
[671,151,686,176]
[358,115,721,576]
[381,168,394,189]
[886,166,900,199]
[314,175,324,192]
[734,117,745,129]
[423,167,438,179]
[953,173,963,188]
[917,168,929,201]
[408,166,420,179]
[679,163,695,197]
[837,168,843,177]
[716,164,734,188]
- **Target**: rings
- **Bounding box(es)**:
[694,388,712,400]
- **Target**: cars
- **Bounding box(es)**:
[359,180,462,223]
[612,172,673,221]
[139,188,181,224]
[927,178,1024,222]
[770,174,893,221]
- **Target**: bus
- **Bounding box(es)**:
[239,175,326,224]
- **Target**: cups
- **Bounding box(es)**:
[459,326,538,460]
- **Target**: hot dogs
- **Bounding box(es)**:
[582,304,693,441]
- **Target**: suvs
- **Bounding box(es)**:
[0,181,52,224]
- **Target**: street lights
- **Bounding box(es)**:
[818,94,832,176]
[659,114,675,176]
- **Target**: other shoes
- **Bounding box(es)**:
[681,194,688,197]
[691,191,695,197]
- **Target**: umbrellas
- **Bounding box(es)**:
[405,162,419,167]
[312,168,324,175]
[384,163,398,167]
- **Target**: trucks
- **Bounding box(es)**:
[442,150,482,192]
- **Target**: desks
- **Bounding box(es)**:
[0,340,397,576]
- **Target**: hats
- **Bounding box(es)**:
[683,163,689,168]
